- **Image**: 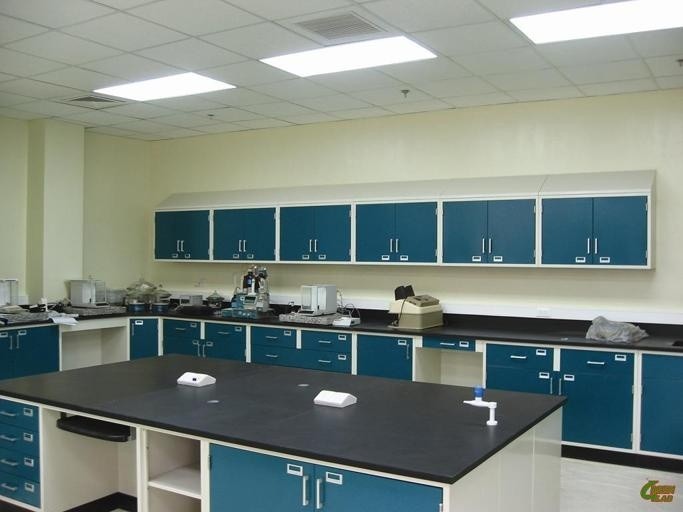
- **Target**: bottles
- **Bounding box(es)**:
[206,289,225,315]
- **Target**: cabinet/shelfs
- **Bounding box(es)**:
[0,325,63,378]
[202,442,441,512]
[0,397,46,512]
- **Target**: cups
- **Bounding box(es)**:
[151,303,169,315]
[127,302,146,314]
